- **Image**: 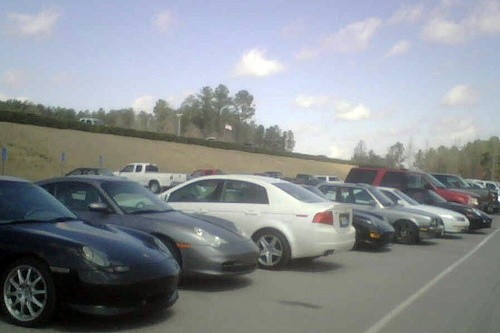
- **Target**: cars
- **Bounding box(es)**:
[1,176,181,327]
[403,187,492,230]
[315,182,443,243]
[159,174,357,269]
[190,169,225,178]
[35,174,260,278]
[436,175,500,213]
[379,186,471,233]
[64,168,113,175]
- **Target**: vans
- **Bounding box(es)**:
[260,171,285,179]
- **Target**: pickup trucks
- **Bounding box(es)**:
[312,175,344,183]
[113,163,187,193]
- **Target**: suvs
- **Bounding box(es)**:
[344,168,482,209]
